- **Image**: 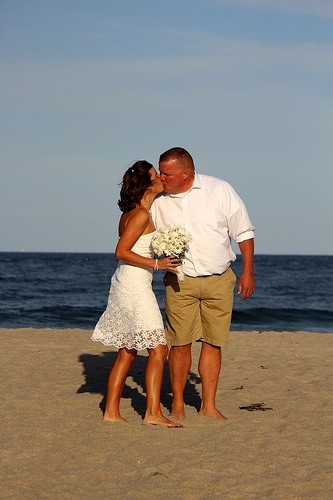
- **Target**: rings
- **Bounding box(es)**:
[251,290,253,293]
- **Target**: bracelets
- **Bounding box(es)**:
[154,259,159,270]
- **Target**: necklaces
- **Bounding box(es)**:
[141,201,151,214]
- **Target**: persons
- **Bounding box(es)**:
[149,148,255,421]
[91,160,183,428]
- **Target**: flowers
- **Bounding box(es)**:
[151,224,192,281]
